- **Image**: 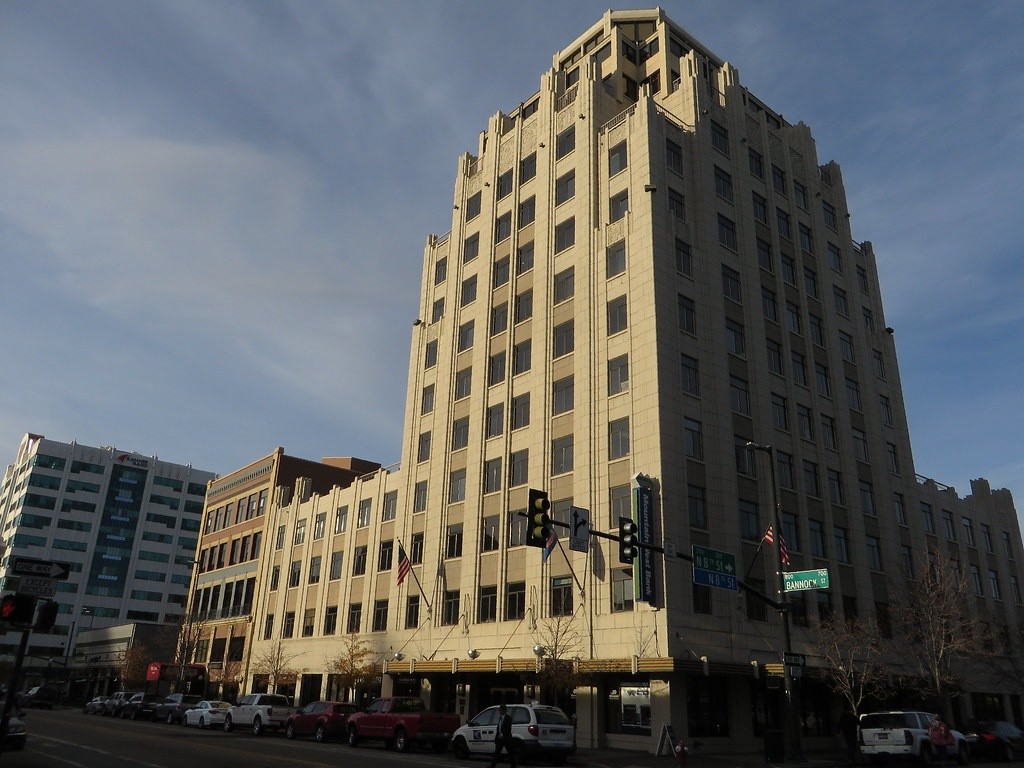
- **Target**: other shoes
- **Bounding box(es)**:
[486,765,495,768]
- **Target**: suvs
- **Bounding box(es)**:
[856,711,970,768]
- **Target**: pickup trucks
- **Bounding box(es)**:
[345,697,461,753]
[224,693,301,735]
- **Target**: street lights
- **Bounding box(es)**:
[746,442,801,761]
[177,560,202,693]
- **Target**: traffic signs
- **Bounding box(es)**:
[783,652,806,667]
[691,544,737,591]
[13,558,71,579]
[569,506,589,553]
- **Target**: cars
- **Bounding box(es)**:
[286,701,359,744]
[83,692,164,721]
[0,686,58,751]
[183,700,233,729]
[150,693,204,725]
[450,704,575,765]
[958,721,1024,762]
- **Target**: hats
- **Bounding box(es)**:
[497,705,509,712]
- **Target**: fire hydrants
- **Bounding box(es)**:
[675,737,689,768]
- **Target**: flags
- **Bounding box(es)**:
[543,527,558,562]
[762,522,792,566]
[397,544,411,586]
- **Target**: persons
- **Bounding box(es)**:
[835,711,861,763]
[488,703,516,768]
[928,710,956,768]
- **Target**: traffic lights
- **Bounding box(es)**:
[526,489,550,548]
[1,595,26,622]
[619,516,637,564]
